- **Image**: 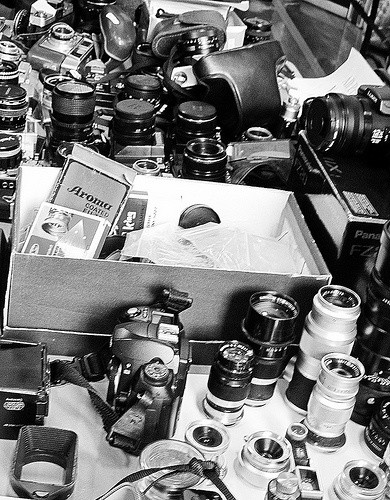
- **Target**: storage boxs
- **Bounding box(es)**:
[0,163,334,346]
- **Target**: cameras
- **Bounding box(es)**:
[307,84,390,168]
[108,287,194,452]
[27,22,94,76]
